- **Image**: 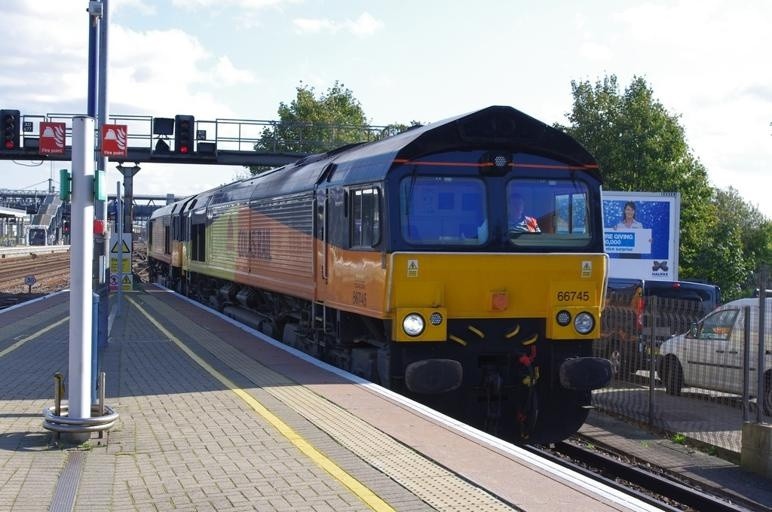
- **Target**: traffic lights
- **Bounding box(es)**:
[0,110,20,151]
[175,115,194,155]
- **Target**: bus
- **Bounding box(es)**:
[594,277,721,380]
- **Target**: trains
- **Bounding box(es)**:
[147,105,614,444]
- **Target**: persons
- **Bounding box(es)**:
[505,192,543,235]
[613,201,644,231]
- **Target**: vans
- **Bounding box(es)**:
[656,288,772,417]
